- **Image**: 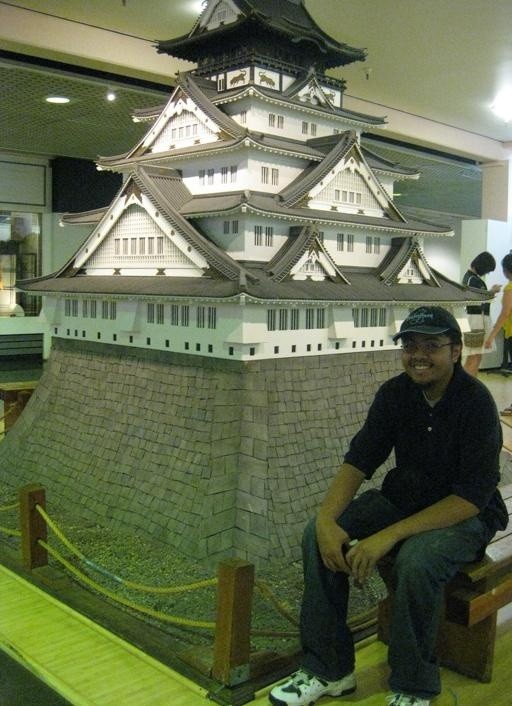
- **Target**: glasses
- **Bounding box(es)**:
[400,340,458,353]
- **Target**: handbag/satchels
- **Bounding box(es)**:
[464,328,486,348]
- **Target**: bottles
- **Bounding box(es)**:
[349,538,388,602]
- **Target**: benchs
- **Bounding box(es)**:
[372,484,511,683]
[1,380,38,403]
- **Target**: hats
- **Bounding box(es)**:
[393,306,461,342]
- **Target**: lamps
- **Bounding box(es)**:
[107,88,115,102]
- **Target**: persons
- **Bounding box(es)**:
[264,304,508,706]
[460,249,502,378]
[483,253,512,374]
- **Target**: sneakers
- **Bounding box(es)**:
[269,666,356,706]
[388,694,430,706]
